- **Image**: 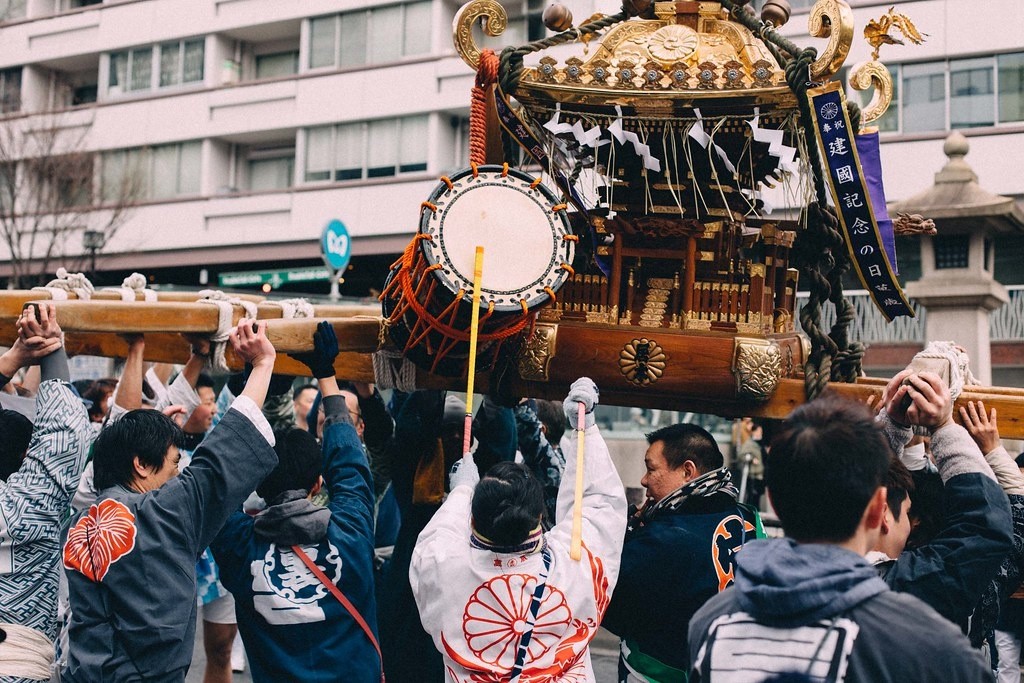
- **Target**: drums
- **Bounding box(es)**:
[379,162,577,368]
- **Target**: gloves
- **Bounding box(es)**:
[287,322,337,379]
[562,379,598,432]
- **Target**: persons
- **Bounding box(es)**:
[686,401,1007,683]
[0,304,1024,683]
